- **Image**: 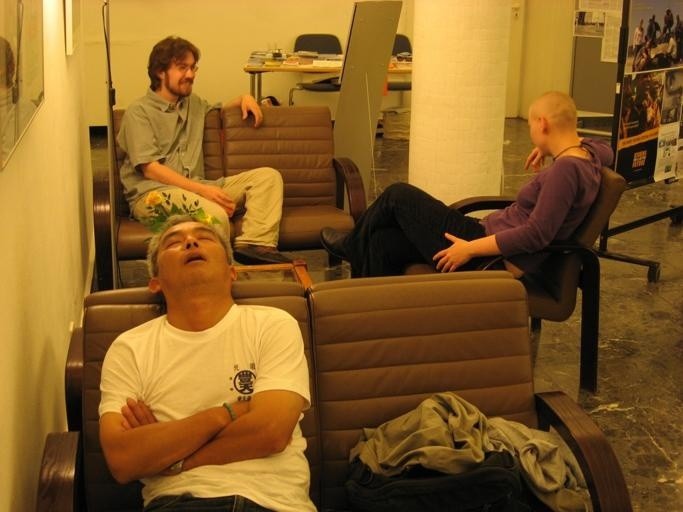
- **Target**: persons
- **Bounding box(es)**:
[117,34,291,263]
[319,90,615,281]
[99,212,320,512]
[619,7,683,140]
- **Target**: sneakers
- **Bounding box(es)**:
[233,243,293,265]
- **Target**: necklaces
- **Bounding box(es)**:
[552,143,584,161]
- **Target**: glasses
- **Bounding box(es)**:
[175,65,199,73]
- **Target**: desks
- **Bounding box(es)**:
[246,53,413,110]
[235,258,312,286]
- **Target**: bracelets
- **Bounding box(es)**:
[222,401,238,421]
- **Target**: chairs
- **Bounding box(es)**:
[36,271,629,512]
[285,35,413,111]
[89,104,367,290]
[403,167,626,391]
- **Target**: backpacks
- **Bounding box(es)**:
[345,449,519,512]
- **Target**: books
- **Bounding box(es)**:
[246,48,412,67]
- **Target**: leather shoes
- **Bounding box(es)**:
[320,227,350,261]
